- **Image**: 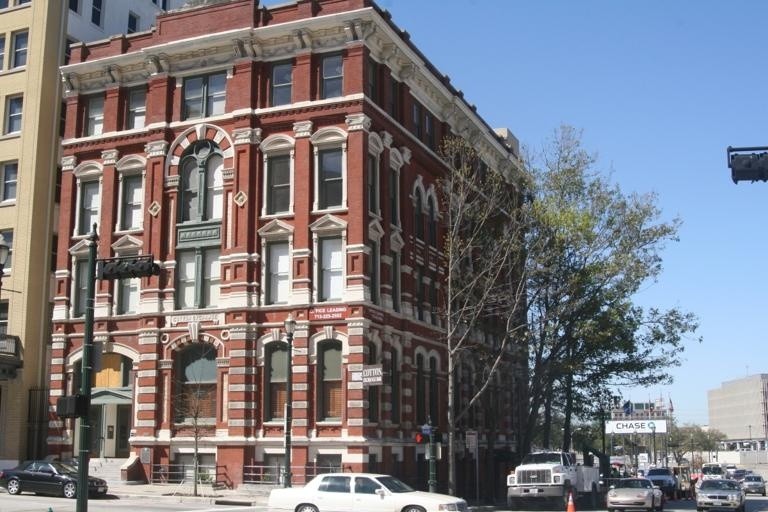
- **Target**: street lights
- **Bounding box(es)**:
[650,424,656,462]
[283,312,297,488]
[610,430,615,456]
[632,428,637,463]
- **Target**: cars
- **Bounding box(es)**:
[0,460,108,499]
[606,463,767,512]
[267,472,468,512]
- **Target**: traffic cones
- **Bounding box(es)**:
[567,491,576,512]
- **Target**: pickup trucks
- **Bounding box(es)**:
[506,451,599,512]
